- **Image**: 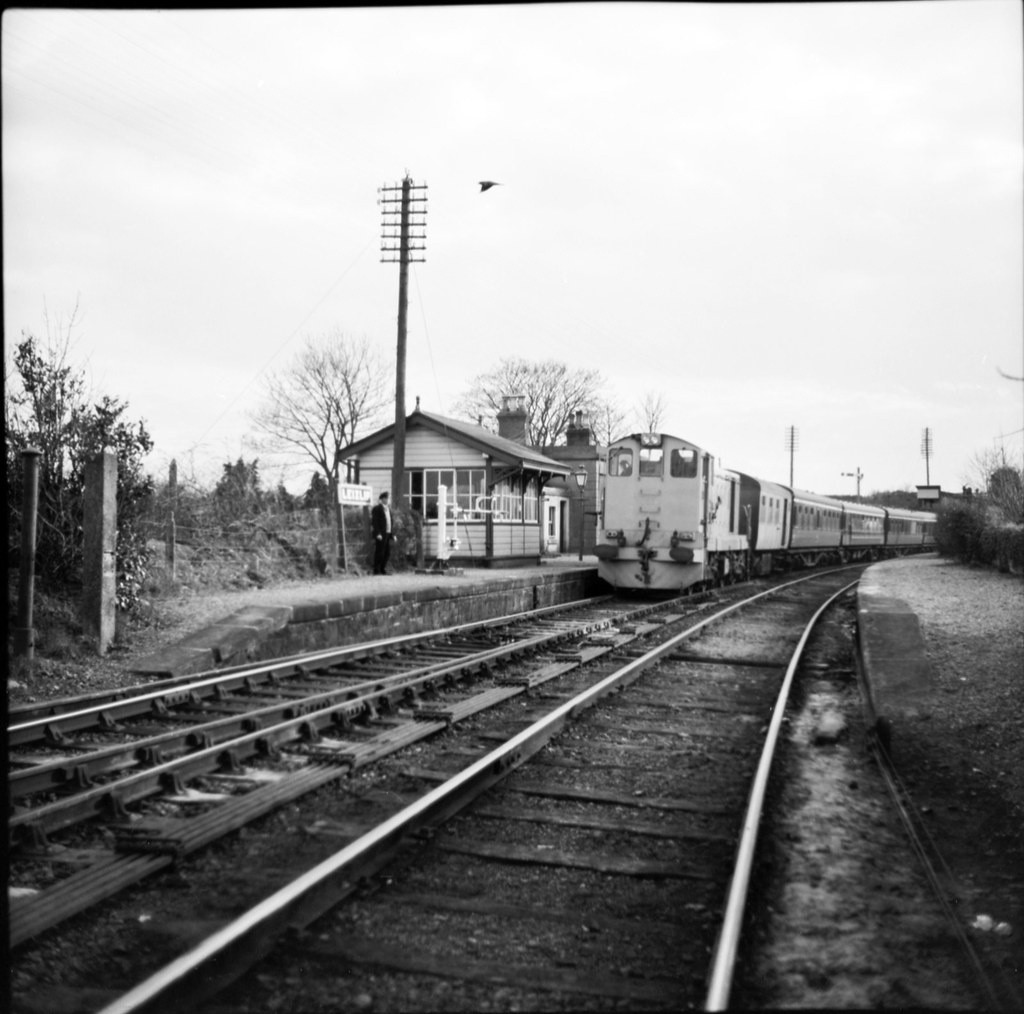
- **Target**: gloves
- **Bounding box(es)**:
[392,535,397,542]
[376,534,382,541]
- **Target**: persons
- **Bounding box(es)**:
[371,492,397,576]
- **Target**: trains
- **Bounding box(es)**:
[592,432,938,598]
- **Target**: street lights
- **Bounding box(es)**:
[574,465,588,563]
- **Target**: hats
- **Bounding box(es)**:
[380,492,388,499]
